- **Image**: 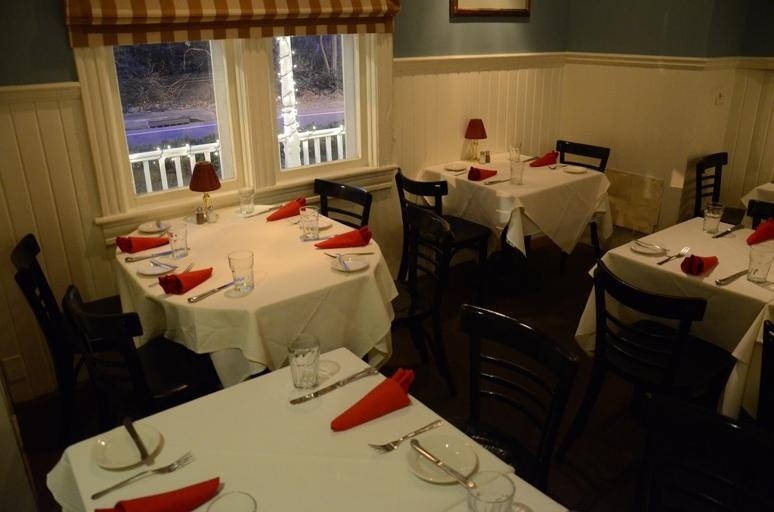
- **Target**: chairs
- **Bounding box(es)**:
[384,203,459,399]
[746,199,773,229]
[694,152,728,217]
[568,257,737,510]
[313,178,373,230]
[555,139,610,253]
[11,232,221,431]
[454,303,579,494]
[633,319,774,512]
[394,166,492,296]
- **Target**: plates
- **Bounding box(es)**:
[93,422,162,470]
[330,257,369,272]
[318,219,332,228]
[443,163,466,171]
[631,241,665,254]
[563,166,587,174]
[137,221,170,233]
[137,262,176,276]
[407,438,477,484]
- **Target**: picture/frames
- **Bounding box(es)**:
[449,0,531,18]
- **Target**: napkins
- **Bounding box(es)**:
[680,254,719,277]
[467,166,497,182]
[746,217,773,246]
[157,266,213,296]
[94,477,222,512]
[329,365,416,433]
[314,226,373,250]
[529,150,559,167]
[267,197,306,221]
[115,234,171,254]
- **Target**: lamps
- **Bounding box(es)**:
[465,119,487,161]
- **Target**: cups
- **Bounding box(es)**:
[702,202,726,233]
[208,491,257,512]
[299,205,319,240]
[466,469,515,511]
[287,332,321,391]
[508,140,523,184]
[227,249,254,291]
[167,225,187,259]
[237,185,256,216]
[746,242,773,283]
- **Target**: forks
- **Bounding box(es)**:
[90,451,195,499]
[148,263,193,288]
[366,418,443,452]
[548,164,568,169]
[655,247,690,265]
[323,251,374,258]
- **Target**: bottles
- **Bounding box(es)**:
[479,150,490,164]
[196,207,204,224]
[207,206,216,223]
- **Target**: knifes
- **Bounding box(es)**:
[186,278,243,303]
[409,437,476,489]
[711,224,744,238]
[289,367,379,405]
[522,156,538,163]
[244,205,282,219]
[150,258,178,269]
[715,267,748,285]
[484,178,510,185]
[121,417,154,465]
[125,247,189,263]
